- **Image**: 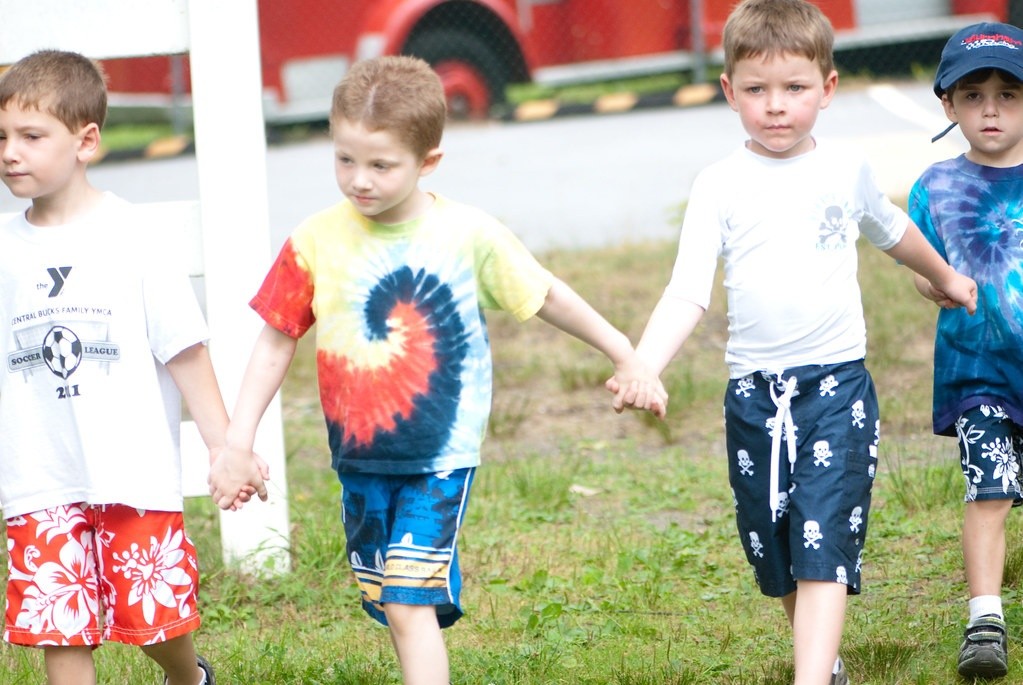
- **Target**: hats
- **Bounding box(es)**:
[932,22,1023,99]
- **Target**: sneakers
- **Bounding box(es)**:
[956,613,1008,676]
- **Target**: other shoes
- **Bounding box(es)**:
[163,654,216,685]
[829,653,850,685]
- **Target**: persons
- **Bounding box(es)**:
[209,54,668,685]
[0,48,269,685]
[604,1,979,685]
[908,21,1023,678]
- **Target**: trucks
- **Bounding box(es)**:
[1,1,1023,121]
